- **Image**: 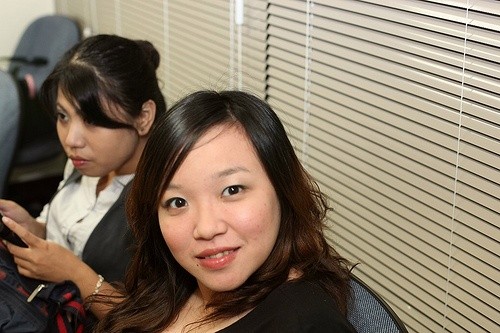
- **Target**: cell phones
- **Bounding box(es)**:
[0,214,29,248]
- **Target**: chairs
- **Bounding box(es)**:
[0,70,21,186]
[8,15,80,183]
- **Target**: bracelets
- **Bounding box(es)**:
[85,274,104,311]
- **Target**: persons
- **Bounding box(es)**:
[0,34,166,333]
[76,90,361,333]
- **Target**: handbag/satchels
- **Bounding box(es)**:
[0,238,86,333]
